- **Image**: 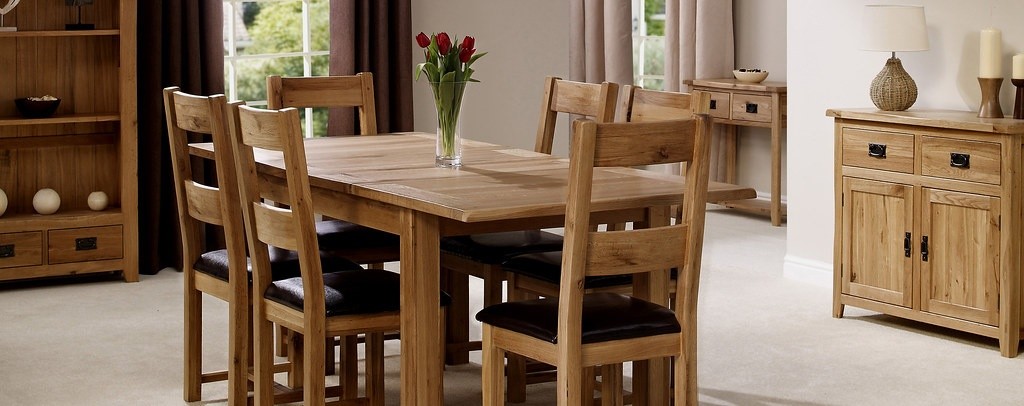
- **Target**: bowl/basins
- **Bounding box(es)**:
[14,97,60,118]
[732,69,769,84]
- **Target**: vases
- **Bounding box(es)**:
[428,82,467,167]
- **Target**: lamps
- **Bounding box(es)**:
[859,3,931,111]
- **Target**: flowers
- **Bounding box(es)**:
[414,31,489,159]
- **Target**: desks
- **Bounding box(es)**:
[188,131,757,406]
[683,79,787,226]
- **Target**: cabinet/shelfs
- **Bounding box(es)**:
[827,104,1024,359]
[0,0,138,285]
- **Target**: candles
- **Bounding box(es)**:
[1012,50,1024,79]
[980,26,1002,78]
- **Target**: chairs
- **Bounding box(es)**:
[227,99,446,406]
[447,78,620,367]
[475,114,715,406]
[505,85,714,400]
[164,86,362,406]
